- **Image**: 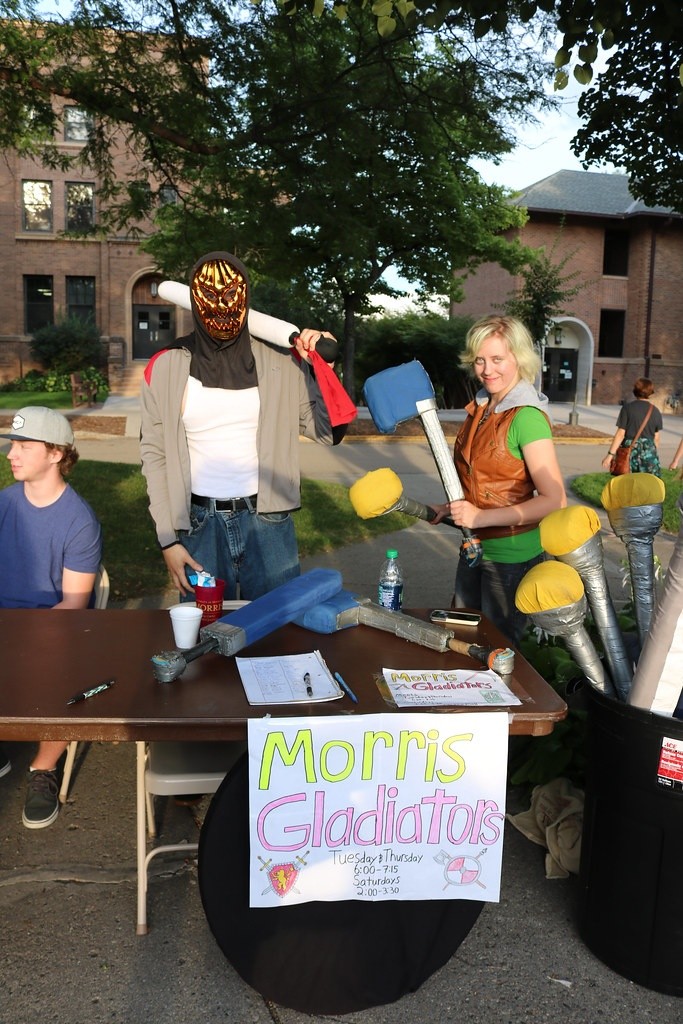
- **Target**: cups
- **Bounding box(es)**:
[169,607,203,650]
[193,578,225,622]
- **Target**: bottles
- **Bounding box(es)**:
[377,549,404,613]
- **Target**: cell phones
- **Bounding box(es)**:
[431,610,482,626]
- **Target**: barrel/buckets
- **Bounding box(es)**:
[567,676,683,997]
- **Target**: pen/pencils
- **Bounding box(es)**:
[303,672,313,696]
[66,681,114,705]
[335,672,358,704]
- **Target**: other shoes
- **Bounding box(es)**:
[173,794,201,806]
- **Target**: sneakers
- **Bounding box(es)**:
[22,764,60,829]
[0,752,11,779]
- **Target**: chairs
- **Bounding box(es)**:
[56,564,111,804]
[137,597,257,937]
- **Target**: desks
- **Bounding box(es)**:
[1,598,569,933]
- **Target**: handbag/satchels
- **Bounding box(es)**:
[610,447,632,476]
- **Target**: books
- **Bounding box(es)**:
[235,650,343,706]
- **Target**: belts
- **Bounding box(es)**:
[191,492,257,511]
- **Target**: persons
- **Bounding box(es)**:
[669,438,683,508]
[602,378,663,479]
[430,313,567,646]
[0,406,102,829]
[140,251,349,603]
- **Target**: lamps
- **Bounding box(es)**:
[151,276,157,297]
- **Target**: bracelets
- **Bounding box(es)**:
[608,451,614,455]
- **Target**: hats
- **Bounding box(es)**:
[0,406,74,449]
